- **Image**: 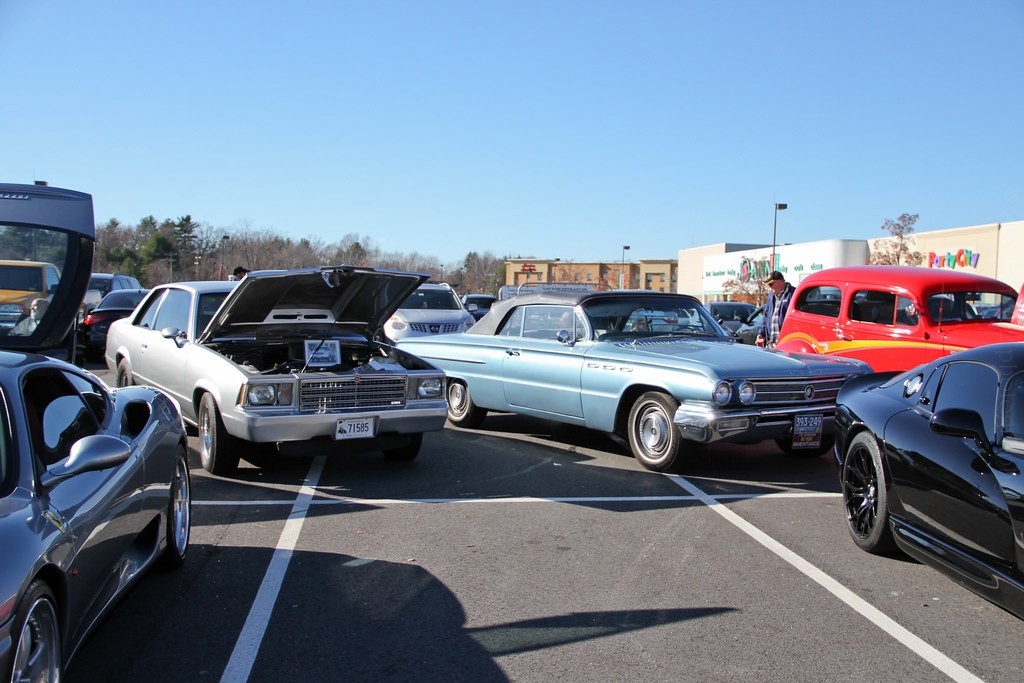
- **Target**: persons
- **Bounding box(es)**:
[755,270,796,348]
[232,267,248,279]
[8,298,52,336]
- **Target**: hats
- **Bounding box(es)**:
[233,266,244,276]
[763,270,784,285]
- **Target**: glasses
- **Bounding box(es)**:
[769,280,778,286]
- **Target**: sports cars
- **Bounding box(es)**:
[837,340,1024,623]
[0,181,193,683]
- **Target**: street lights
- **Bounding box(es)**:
[553,259,560,286]
[440,264,444,283]
[622,246,631,290]
[773,203,787,271]
[195,257,201,281]
[220,235,229,280]
[461,268,463,294]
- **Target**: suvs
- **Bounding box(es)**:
[460,292,498,322]
[383,281,477,343]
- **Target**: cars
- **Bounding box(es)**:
[623,309,691,334]
[854,294,977,323]
[771,265,1024,374]
[735,300,765,347]
[689,301,762,335]
[0,260,62,334]
[78,273,142,322]
[394,290,875,474]
[105,264,450,475]
[970,298,1013,320]
[82,288,154,360]
